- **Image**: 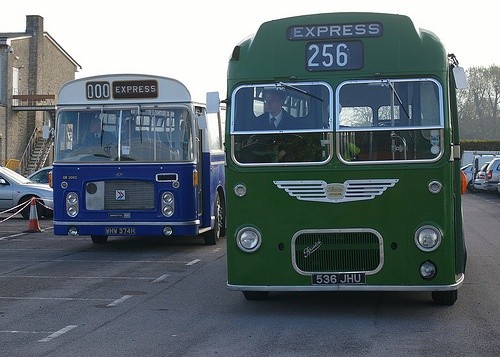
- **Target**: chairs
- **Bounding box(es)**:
[321,125,407,162]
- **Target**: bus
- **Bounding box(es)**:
[43,74,226,245]
[206,12,468,305]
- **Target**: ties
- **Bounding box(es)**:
[271,117,277,130]
[96,133,99,138]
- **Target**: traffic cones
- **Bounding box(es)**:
[23,197,44,233]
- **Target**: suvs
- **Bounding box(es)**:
[461,150,500,194]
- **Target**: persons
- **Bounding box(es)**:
[122,120,147,147]
[244,90,302,160]
[84,118,118,148]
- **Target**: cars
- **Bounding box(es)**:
[0,165,53,219]
